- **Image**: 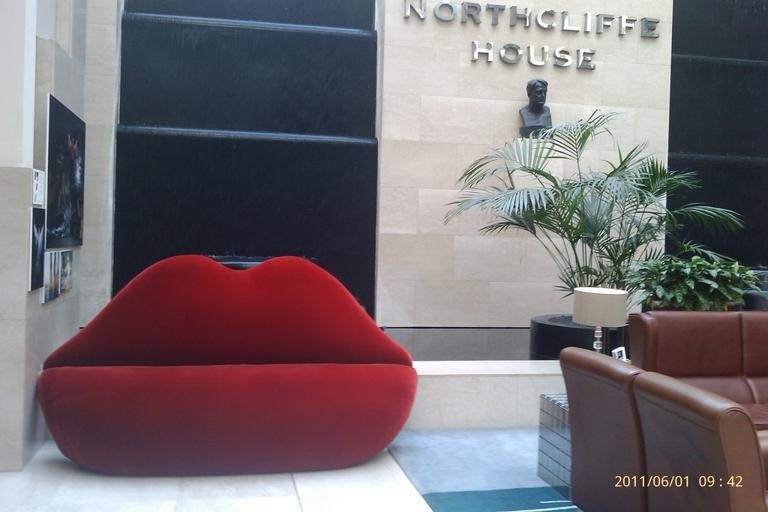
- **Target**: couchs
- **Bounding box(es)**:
[35,254,419,476]
[631,308,756,424]
[562,345,649,511]
[737,309,768,425]
[630,369,766,510]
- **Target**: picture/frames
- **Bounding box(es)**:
[611,346,627,360]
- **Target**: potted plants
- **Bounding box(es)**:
[442,108,746,359]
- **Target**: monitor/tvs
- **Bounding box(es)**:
[43,92,86,253]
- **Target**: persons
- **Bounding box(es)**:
[519,79,553,139]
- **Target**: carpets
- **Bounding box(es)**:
[385,426,580,512]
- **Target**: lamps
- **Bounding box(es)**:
[570,287,629,354]
